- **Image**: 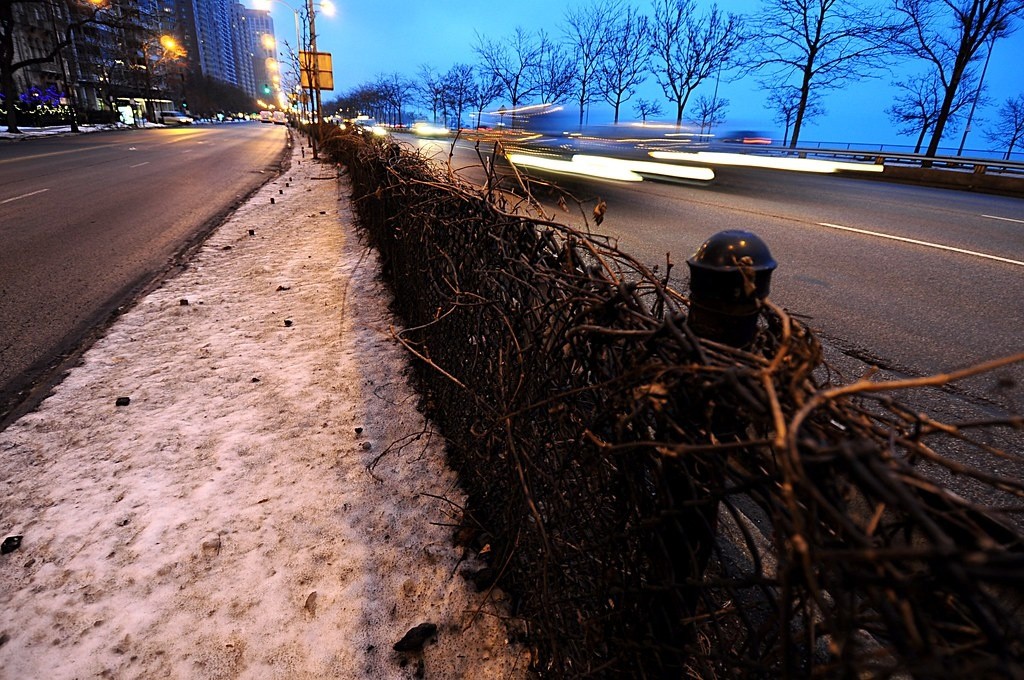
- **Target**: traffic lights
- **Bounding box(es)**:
[264,84,269,93]
[293,100,298,109]
[182,102,187,108]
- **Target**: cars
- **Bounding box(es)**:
[162,111,193,125]
[354,120,376,135]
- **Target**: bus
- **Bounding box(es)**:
[273,112,285,124]
[261,111,273,123]
[119,97,175,121]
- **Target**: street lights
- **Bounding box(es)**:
[499,105,507,131]
[252,0,335,131]
